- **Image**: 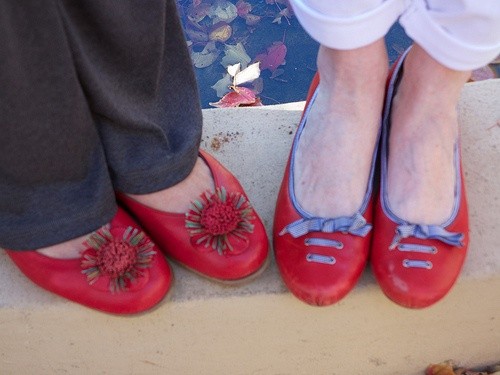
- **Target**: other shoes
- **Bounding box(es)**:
[4,202,173,313]
[370,43,469,309]
[273,70,372,305]
[114,146,271,284]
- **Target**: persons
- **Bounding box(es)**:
[272,1,500,310]
[1,0,272,316]
